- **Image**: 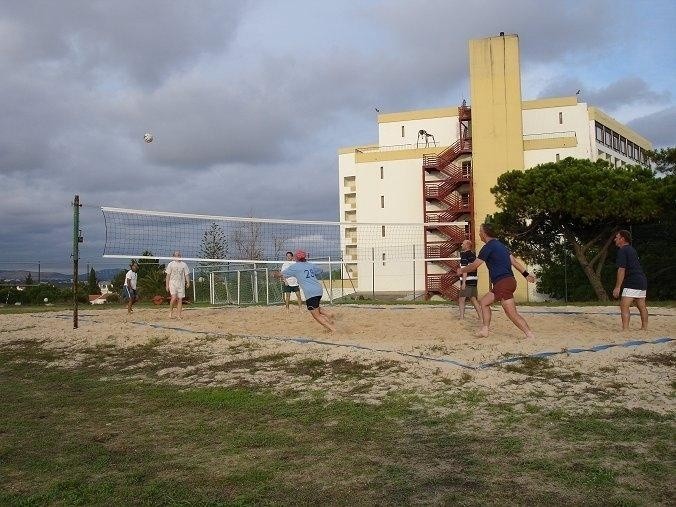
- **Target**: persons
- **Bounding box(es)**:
[612,229,649,331]
[273,248,337,335]
[280,252,303,313]
[456,239,483,323]
[122,262,139,315]
[456,223,537,342]
[165,250,191,319]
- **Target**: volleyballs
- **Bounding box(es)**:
[144,134,154,142]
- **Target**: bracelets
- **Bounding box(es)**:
[521,269,529,278]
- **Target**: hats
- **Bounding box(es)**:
[134,263,139,269]
[296,250,305,261]
